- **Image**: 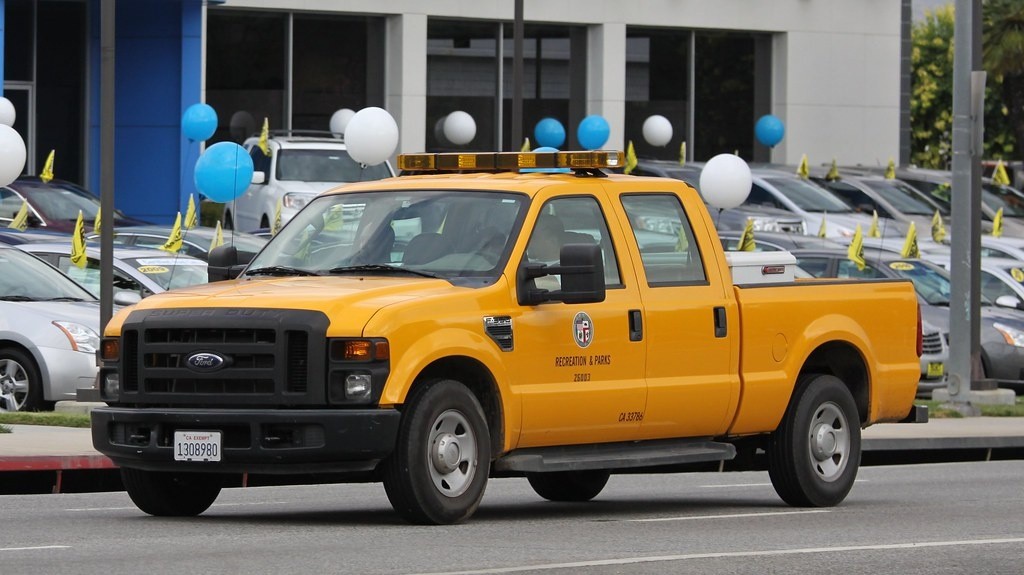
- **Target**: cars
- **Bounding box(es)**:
[785,165,950,238]
[0,241,122,412]
[687,160,911,241]
[874,168,1024,236]
[13,241,210,307]
[785,235,1023,402]
[86,225,263,265]
[0,171,153,232]
[623,157,804,236]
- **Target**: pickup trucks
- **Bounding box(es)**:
[89,151,923,527]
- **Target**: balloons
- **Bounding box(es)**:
[344,107,399,166]
[576,114,610,149]
[330,108,355,138]
[193,142,255,203]
[0,124,26,186]
[534,118,567,148]
[519,146,570,173]
[0,96,16,123]
[755,115,784,146]
[643,115,673,147]
[433,118,451,145]
[181,104,220,142]
[443,111,478,146]
[698,152,753,210]
[231,111,258,141]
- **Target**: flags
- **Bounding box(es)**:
[8,117,1023,299]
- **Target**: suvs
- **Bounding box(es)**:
[223,129,398,241]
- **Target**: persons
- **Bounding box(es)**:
[512,218,575,291]
